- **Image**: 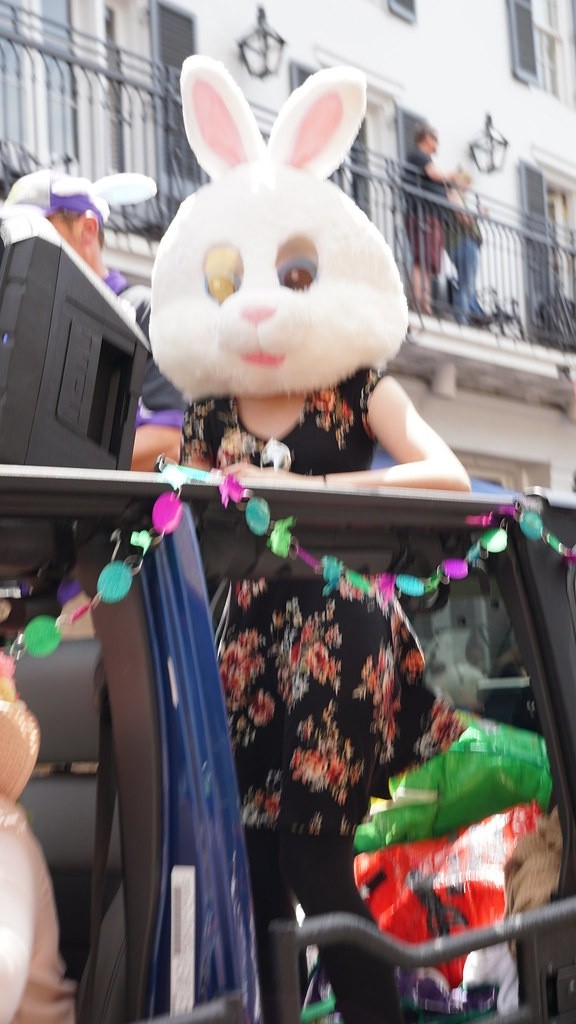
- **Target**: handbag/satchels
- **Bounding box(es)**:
[353,800,543,1017]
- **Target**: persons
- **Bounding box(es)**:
[3,169,190,468]
[404,126,489,324]
[148,53,469,1024]
[0,655,76,1024]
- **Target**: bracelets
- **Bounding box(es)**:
[324,474,327,484]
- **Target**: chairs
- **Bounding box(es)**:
[4,636,124,985]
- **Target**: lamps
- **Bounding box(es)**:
[237,8,285,79]
[472,116,509,175]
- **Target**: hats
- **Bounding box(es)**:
[1,170,110,229]
